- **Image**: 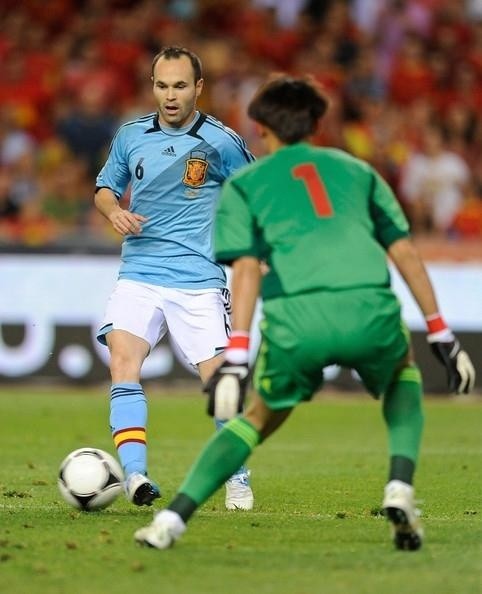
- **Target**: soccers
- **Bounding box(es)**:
[57,447,125,513]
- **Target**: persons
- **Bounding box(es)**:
[131,70,475,551]
[93,45,253,508]
[1,1,481,259]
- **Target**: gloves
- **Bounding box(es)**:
[425,329,476,395]
[203,350,250,420]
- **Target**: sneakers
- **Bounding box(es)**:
[126,475,161,505]
[378,479,422,550]
[133,509,185,550]
[224,472,255,512]
